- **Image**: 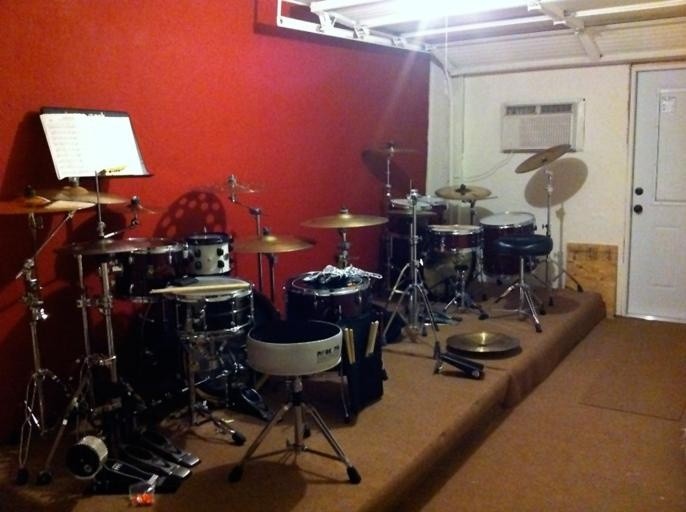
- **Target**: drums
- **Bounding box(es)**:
[428,224,483,253]
[479,212,536,275]
[113,237,185,304]
[140,289,284,402]
[163,277,253,344]
[283,272,373,321]
[389,199,446,240]
[418,196,448,224]
[185,232,232,276]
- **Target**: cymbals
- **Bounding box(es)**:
[193,180,266,195]
[53,240,149,254]
[515,145,570,173]
[36,187,130,204]
[365,147,415,154]
[435,186,491,200]
[1,196,95,214]
[233,236,315,253]
[445,331,519,353]
[300,214,388,228]
[389,210,435,216]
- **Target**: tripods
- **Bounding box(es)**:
[383,215,438,343]
[442,265,482,314]
[12,266,124,487]
[154,306,249,446]
[530,185,582,308]
